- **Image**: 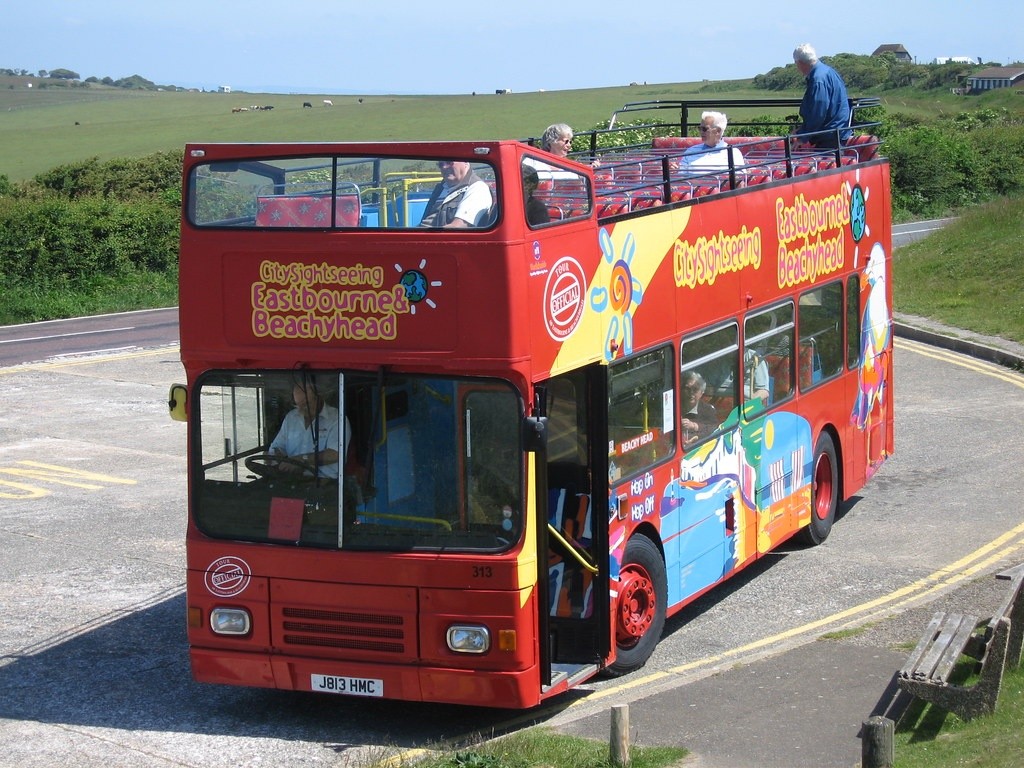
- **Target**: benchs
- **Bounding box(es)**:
[256,133,882,229]
[701,395,735,425]
[640,428,670,469]
[765,335,813,400]
[900,564,1024,722]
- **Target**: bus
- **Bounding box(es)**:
[168,97,894,708]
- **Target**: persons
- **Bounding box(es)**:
[747,319,789,356]
[266,378,351,490]
[677,111,748,175]
[519,122,603,180]
[416,160,492,227]
[793,44,855,146]
[703,333,770,402]
[487,164,552,226]
[680,369,721,444]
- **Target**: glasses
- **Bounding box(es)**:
[562,138,574,145]
[292,403,309,409]
[436,161,454,167]
[697,125,718,134]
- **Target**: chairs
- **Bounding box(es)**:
[342,399,379,490]
